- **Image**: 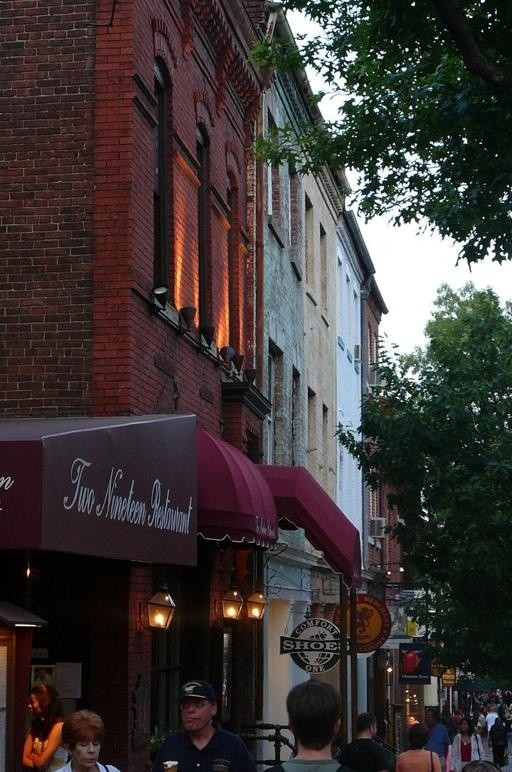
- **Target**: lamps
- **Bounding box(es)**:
[247,589,269,626]
[138,581,177,632]
[368,562,405,576]
[213,585,246,627]
[149,285,257,388]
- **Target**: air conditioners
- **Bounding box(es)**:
[368,516,389,539]
[353,343,361,364]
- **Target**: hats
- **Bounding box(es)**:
[179,679,216,704]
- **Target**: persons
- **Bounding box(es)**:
[262,677,357,771]
[54,709,123,771]
[152,677,257,772]
[339,711,388,772]
[395,722,442,772]
[424,688,511,771]
[22,680,70,771]
